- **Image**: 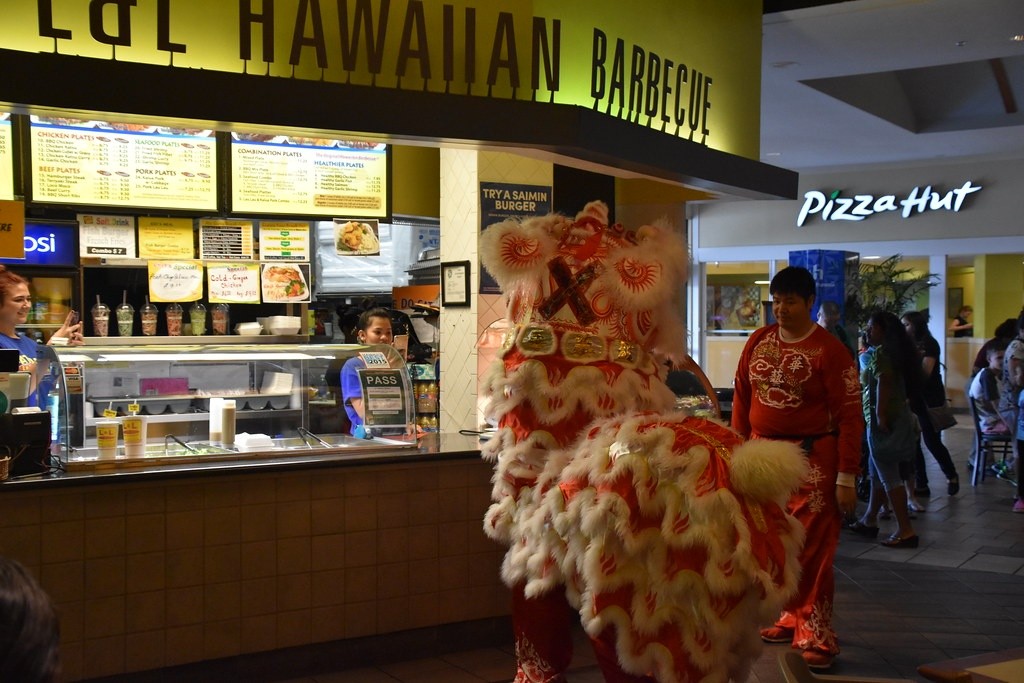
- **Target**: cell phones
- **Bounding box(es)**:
[69,311,79,342]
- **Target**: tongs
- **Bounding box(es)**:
[164,433,198,456]
[297,427,334,449]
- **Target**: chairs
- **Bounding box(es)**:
[967,395,1020,488]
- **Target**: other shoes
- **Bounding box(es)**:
[968,463,974,471]
[803,650,836,667]
[913,487,931,497]
[907,508,917,520]
[1012,500,1024,512]
[761,627,795,642]
[991,461,1006,472]
[947,474,959,495]
[908,502,925,512]
[880,510,891,520]
[996,466,1018,486]
[985,468,994,476]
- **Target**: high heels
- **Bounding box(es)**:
[847,521,880,538]
[881,535,919,548]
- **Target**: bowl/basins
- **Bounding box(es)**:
[234,315,302,335]
[234,433,275,452]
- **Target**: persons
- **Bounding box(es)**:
[968,306,1024,513]
[817,302,961,551]
[341,310,423,437]
[0,264,83,464]
[947,304,974,337]
[729,264,861,668]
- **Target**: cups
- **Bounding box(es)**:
[0,459,9,480]
[120,415,149,457]
[189,304,207,336]
[91,303,111,337]
[210,304,229,335]
[165,304,184,336]
[94,421,120,460]
[324,323,333,336]
[139,304,159,336]
[115,304,136,336]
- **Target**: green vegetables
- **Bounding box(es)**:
[337,238,352,251]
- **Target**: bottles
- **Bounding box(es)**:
[318,375,328,402]
[413,382,438,433]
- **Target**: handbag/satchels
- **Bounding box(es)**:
[870,408,919,464]
[928,403,958,433]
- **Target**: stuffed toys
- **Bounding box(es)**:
[476,199,814,683]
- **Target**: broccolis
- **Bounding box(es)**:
[285,279,305,296]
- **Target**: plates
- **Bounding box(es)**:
[335,223,380,255]
[263,264,310,301]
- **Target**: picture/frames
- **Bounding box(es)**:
[442,260,472,308]
